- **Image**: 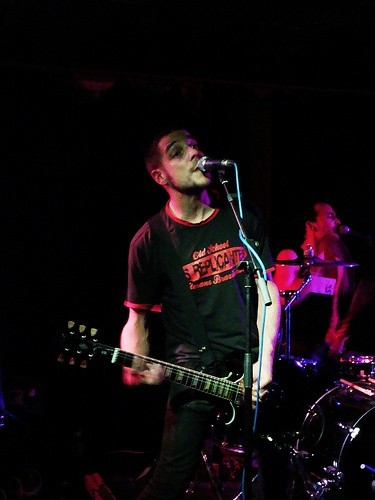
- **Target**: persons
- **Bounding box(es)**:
[119,124,282,500]
[273,201,349,349]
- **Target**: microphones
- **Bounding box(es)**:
[339,225,372,244]
[198,155,233,172]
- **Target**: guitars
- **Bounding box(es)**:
[57,319,244,427]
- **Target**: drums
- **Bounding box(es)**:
[295,381,375,500]
[336,350,374,378]
